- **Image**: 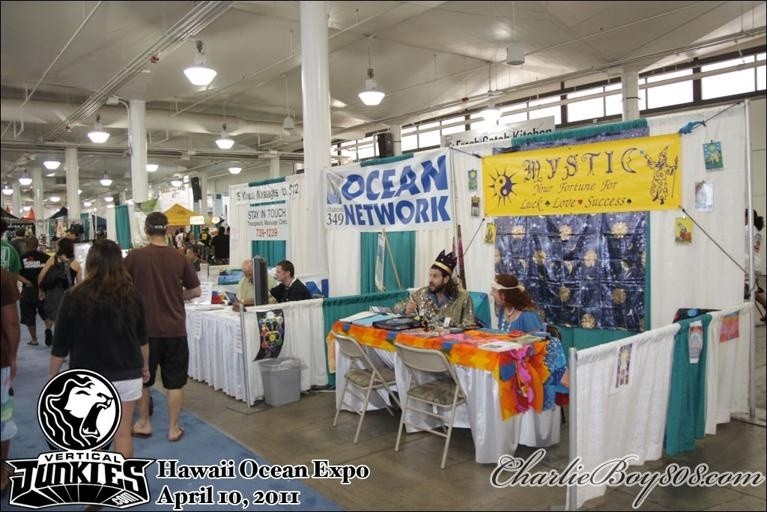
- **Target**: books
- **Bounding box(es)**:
[339,305,523,354]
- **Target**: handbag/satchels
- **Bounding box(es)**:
[40,262,67,291]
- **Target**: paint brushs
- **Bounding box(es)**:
[409,295,420,321]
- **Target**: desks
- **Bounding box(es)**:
[331,309,551,466]
[183,303,251,399]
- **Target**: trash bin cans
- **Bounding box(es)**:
[258,357,303,407]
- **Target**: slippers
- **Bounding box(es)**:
[169,428,183,442]
[27,341,39,345]
[131,424,151,438]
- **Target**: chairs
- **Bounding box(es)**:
[670,308,720,327]
[329,332,402,446]
[544,324,569,425]
[473,316,488,328]
[393,339,468,469]
[310,293,325,299]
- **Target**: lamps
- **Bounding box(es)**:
[0,0,525,213]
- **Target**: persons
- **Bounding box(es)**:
[235,258,278,310]
[265,260,312,305]
[400,264,476,329]
[0,218,230,504]
[491,272,545,334]
[745,206,766,321]
[122,210,201,441]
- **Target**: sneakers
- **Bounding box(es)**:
[45,329,52,346]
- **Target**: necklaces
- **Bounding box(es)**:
[506,307,515,317]
